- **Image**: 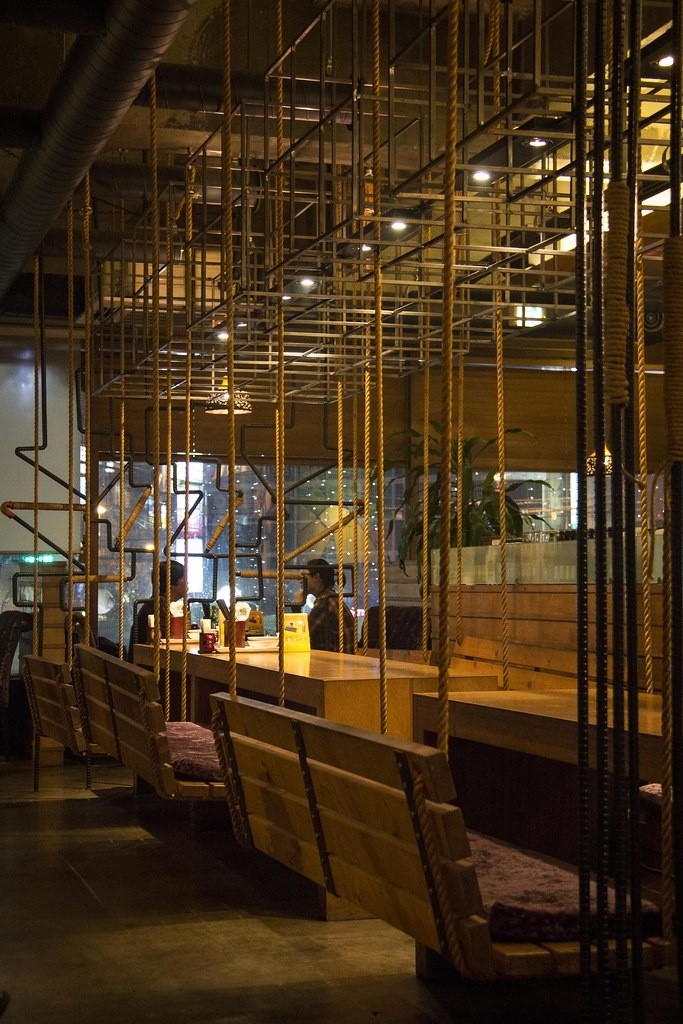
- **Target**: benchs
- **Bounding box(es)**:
[71,643,228,845]
[451,634,628,691]
[22,653,109,759]
[209,690,683,1024]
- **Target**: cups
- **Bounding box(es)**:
[170,616,184,638]
[198,632,216,654]
[224,621,245,648]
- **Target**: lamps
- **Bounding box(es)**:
[649,54,674,68]
[586,443,612,477]
[522,128,554,149]
[204,376,254,415]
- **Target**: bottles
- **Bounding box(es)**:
[210,605,219,643]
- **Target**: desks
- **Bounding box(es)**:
[132,635,279,722]
[408,687,683,1024]
[192,650,498,740]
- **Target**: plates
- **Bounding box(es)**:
[247,636,278,646]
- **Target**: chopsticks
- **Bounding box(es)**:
[215,599,234,621]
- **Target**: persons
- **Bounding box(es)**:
[293,557,355,652]
[126,557,188,674]
